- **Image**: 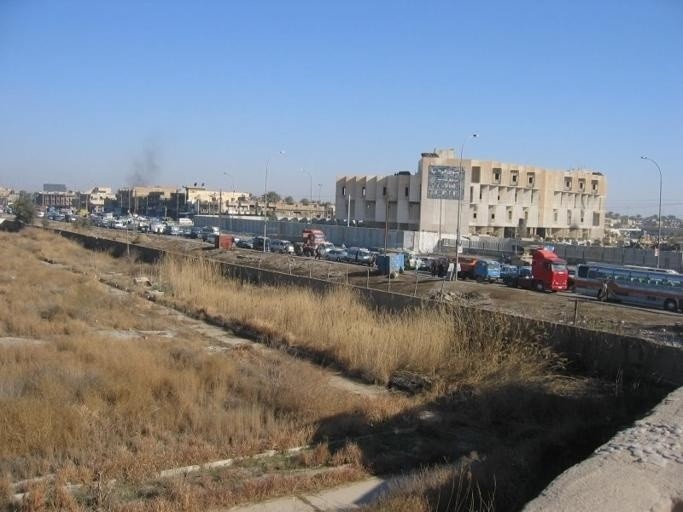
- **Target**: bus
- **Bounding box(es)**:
[573,261,682,312]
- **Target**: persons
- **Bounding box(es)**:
[431,257,461,281]
[314,247,320,260]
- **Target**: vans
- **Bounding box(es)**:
[501,264,517,278]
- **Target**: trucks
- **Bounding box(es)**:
[501,248,568,294]
[449,258,502,285]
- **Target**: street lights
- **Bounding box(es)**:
[262,151,285,252]
[640,155,662,268]
[452,133,478,280]
